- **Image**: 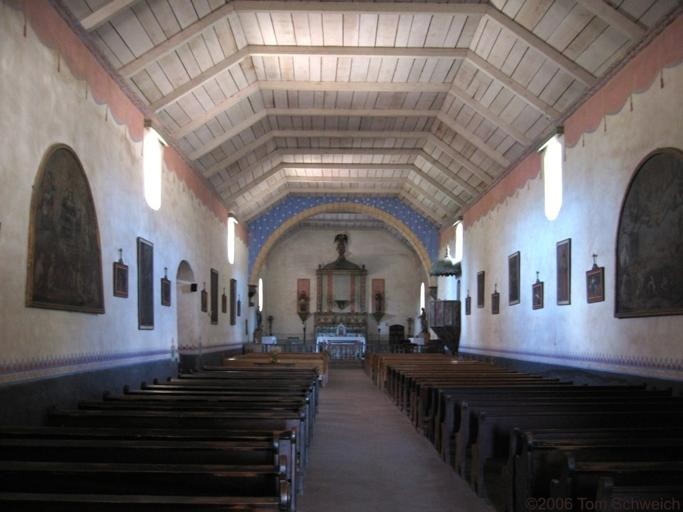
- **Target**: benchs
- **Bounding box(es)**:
[1,350,329,511]
[363,351,683,512]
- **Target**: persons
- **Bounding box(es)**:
[418,308,426,331]
[298,290,307,312]
[375,290,383,312]
[256,305,262,330]
[336,237,346,260]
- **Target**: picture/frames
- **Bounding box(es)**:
[110,237,241,330]
[457,237,604,315]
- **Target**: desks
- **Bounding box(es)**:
[315,337,366,353]
